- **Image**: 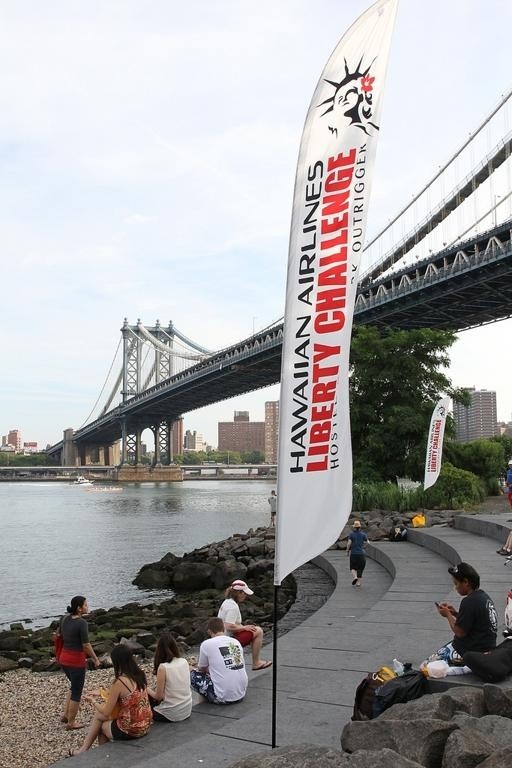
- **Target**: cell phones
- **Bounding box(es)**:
[435,601,441,606]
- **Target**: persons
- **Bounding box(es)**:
[58,595,102,730]
[268,490,276,528]
[68,643,155,757]
[346,520,370,587]
[190,616,250,706]
[495,527,511,555]
[146,634,193,723]
[505,457,512,522]
[218,579,272,671]
[417,562,498,676]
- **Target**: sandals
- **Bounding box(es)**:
[68,748,79,757]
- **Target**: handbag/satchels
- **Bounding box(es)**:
[54,615,65,663]
[350,667,427,721]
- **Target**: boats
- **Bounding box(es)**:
[74,477,95,484]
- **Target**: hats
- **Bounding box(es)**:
[354,521,361,528]
[230,579,254,596]
[508,460,512,465]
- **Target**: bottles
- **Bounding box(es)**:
[393,659,404,676]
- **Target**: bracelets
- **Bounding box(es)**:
[92,701,97,705]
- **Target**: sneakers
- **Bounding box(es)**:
[496,547,511,555]
[351,577,361,587]
[60,716,84,730]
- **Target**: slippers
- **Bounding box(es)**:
[252,661,272,670]
